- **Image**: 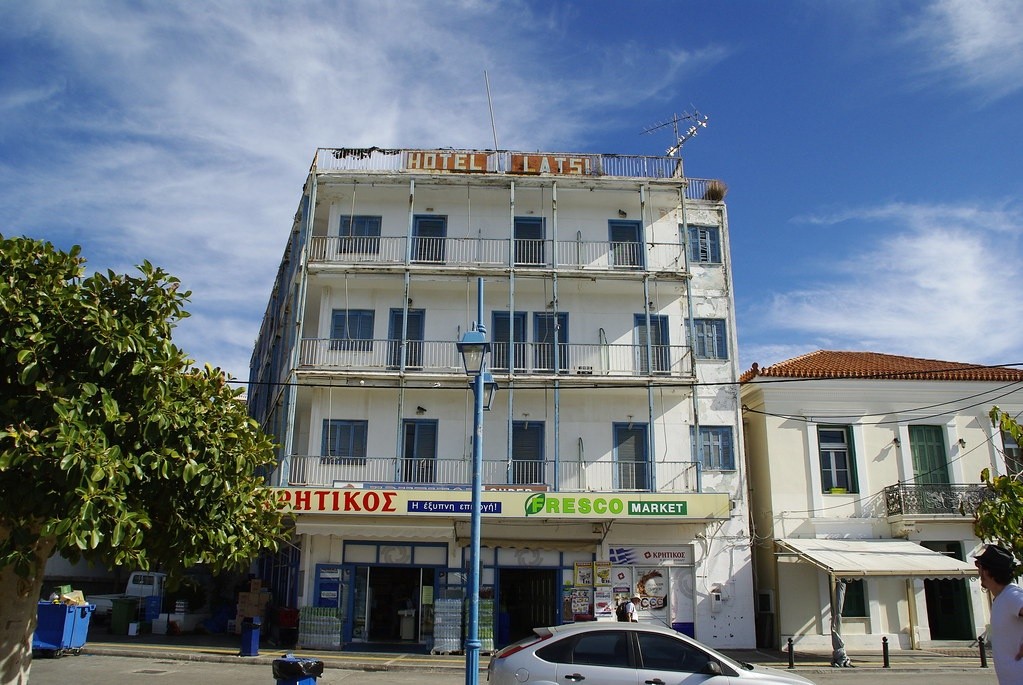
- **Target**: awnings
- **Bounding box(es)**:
[774,538,980,580]
[295,516,454,538]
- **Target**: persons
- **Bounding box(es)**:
[973,544,1023,685]
[390,587,408,639]
[616,592,642,623]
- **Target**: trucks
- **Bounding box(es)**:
[85,571,167,628]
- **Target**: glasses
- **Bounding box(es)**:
[975,561,988,571]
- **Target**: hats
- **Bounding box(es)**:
[630,593,642,601]
[973,544,1014,572]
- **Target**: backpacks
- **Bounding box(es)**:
[616,602,634,621]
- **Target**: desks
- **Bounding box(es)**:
[170,613,215,634]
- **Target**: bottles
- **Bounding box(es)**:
[434,600,461,650]
[298,607,343,646]
[479,601,493,651]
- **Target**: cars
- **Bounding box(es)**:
[487,622,817,685]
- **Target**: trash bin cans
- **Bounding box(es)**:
[31,600,97,656]
[272,653,325,685]
[145,596,161,624]
[110,598,136,635]
[240,616,261,656]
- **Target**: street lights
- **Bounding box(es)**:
[456,322,499,684]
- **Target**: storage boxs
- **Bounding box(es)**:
[236,580,274,637]
